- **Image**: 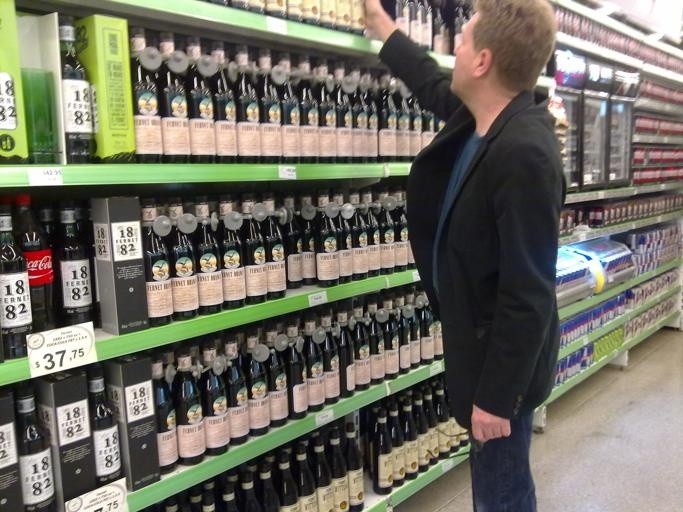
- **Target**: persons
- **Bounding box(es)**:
[353,1,568,510]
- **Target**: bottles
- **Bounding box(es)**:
[550,6,683,72]
[629,67,683,186]
[56,24,98,165]
[126,20,446,165]
[0,187,470,511]
[208,0,466,55]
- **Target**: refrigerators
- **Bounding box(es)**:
[548,49,587,191]
[582,57,609,191]
[605,61,643,189]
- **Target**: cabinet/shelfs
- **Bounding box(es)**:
[0,2,474,512]
[536,0,683,432]
